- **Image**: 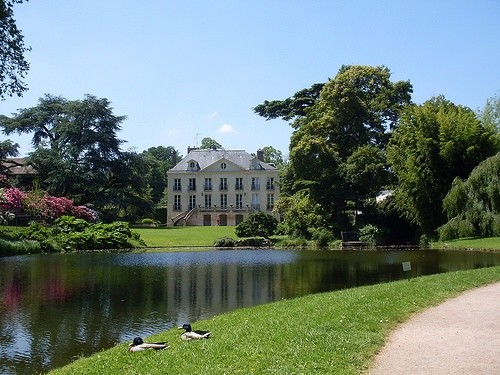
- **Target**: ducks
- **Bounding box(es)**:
[177,324,213,340]
[128,337,171,353]
